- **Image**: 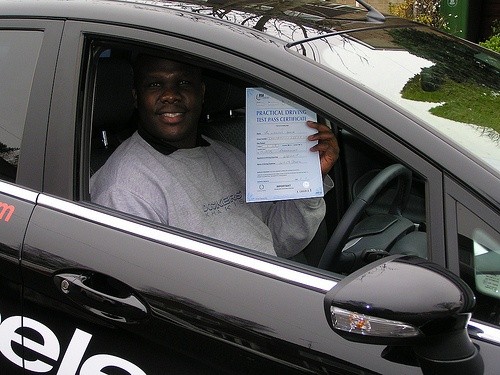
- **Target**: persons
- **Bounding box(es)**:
[89,53,340,257]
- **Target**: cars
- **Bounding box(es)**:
[0,0,500,375]
